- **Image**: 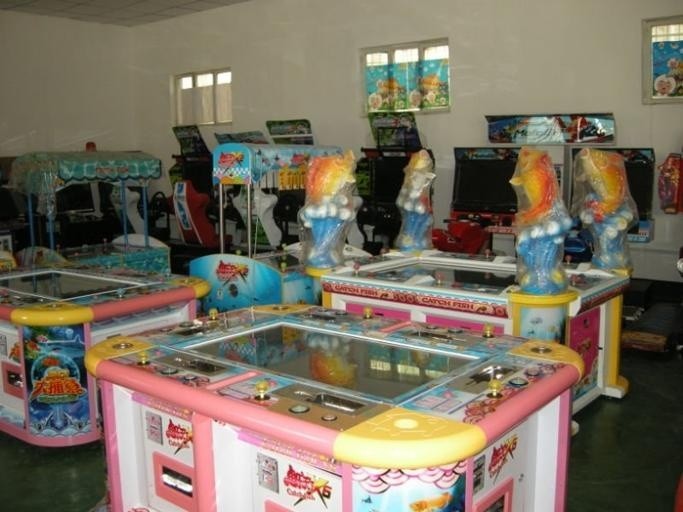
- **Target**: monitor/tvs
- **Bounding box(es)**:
[453,161,519,209]
[54,184,96,216]
[370,157,406,206]
